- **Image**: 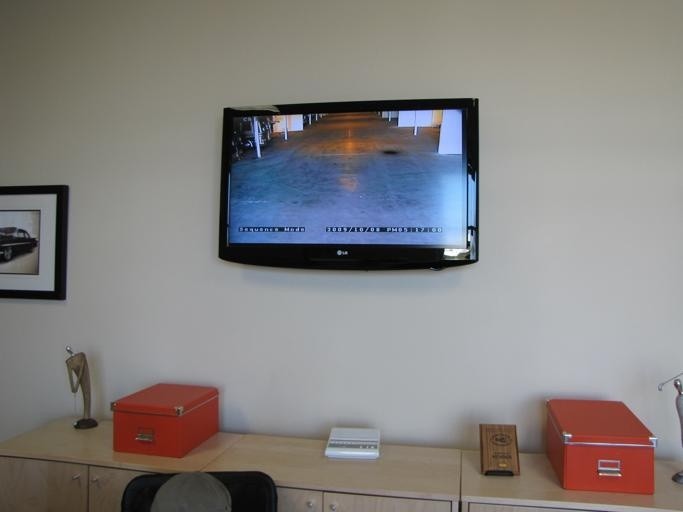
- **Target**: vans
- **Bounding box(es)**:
[235,116,272,151]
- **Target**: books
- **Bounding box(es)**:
[325,427,381,461]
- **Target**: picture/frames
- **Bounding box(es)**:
[0,181,70,302]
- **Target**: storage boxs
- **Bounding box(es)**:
[543,394,658,497]
[104,379,223,462]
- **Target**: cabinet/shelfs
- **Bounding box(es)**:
[456,446,681,512]
[196,429,460,511]
[0,410,241,512]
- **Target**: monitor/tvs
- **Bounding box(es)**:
[219,97,479,270]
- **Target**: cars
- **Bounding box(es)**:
[0,226,39,263]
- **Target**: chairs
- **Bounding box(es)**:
[120,467,278,511]
